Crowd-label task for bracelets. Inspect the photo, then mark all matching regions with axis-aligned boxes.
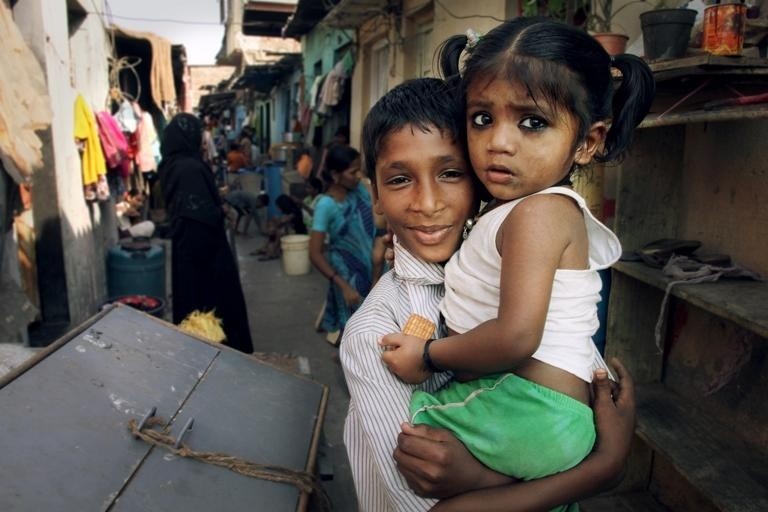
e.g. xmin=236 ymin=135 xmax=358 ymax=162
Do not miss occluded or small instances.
xmin=329 ymin=271 xmax=338 ymax=283
xmin=419 ymin=337 xmax=446 ymax=375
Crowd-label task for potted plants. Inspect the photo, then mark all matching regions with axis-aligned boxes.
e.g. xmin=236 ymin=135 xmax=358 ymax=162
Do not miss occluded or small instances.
xmin=639 ymin=0 xmax=699 ymax=63
xmin=586 ymin=0 xmax=639 ymax=55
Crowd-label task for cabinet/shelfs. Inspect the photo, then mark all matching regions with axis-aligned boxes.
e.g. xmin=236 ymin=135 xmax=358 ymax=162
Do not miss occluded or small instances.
xmin=602 ymin=105 xmax=768 ymax=512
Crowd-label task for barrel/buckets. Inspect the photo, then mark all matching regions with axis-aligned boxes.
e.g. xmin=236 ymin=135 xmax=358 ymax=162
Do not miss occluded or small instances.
xmin=278 ymin=234 xmax=311 ymax=276
xmin=109 ymin=237 xmax=167 ymax=300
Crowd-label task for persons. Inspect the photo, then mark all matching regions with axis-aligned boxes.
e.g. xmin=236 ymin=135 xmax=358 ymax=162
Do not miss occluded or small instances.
xmin=376 ymin=13 xmax=657 ymax=511
xmin=107 ymin=106 xmax=354 ymax=264
xmin=303 ymin=142 xmax=385 ymax=342
xmin=153 ymin=113 xmax=257 ymax=357
xmin=337 ymin=75 xmax=640 ymax=512
